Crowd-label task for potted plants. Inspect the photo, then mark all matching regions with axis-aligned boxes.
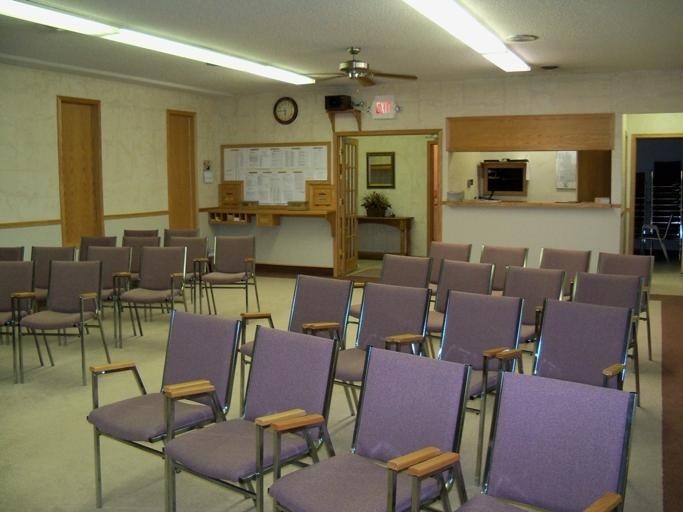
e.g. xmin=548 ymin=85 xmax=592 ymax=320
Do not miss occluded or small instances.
xmin=358 ymin=190 xmax=391 ymax=217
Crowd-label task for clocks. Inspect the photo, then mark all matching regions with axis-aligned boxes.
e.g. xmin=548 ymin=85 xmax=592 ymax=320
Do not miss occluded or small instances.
xmin=272 ymin=97 xmax=298 ymax=125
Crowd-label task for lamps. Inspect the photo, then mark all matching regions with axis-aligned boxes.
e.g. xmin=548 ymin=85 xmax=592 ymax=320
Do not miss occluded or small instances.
xmin=0 ymin=0 xmax=315 ymax=88
xmin=398 ymin=0 xmax=533 ymax=76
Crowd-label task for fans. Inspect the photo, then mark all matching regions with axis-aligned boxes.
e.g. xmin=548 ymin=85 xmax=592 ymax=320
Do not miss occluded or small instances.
xmin=298 ymin=47 xmax=418 ymax=88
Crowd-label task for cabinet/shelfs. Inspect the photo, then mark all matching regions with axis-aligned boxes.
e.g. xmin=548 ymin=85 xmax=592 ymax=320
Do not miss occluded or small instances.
xmin=634 ymin=195 xmax=644 ymax=237
xmin=356 ymin=216 xmax=414 ymax=257
xmin=650 ymin=183 xmax=682 ymax=239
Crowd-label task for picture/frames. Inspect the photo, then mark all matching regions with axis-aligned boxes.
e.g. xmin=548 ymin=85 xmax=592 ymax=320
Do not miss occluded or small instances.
xmin=366 ymin=152 xmax=394 ymax=188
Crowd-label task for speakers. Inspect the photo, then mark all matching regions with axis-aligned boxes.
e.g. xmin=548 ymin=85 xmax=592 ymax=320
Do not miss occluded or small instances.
xmin=324 ymin=95 xmax=352 ymax=111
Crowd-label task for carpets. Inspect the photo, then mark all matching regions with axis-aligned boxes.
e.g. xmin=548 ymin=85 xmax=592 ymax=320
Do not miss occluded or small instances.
xmin=0 ymin=267 xmax=666 ymax=511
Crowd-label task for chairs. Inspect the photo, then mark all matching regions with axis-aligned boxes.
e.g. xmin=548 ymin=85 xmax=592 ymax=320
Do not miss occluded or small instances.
xmin=1 ymin=228 xmax=260 ymax=385
xmin=88 ymin=240 xmax=656 ymax=511
xmin=637 ymin=214 xmax=673 ymax=263
